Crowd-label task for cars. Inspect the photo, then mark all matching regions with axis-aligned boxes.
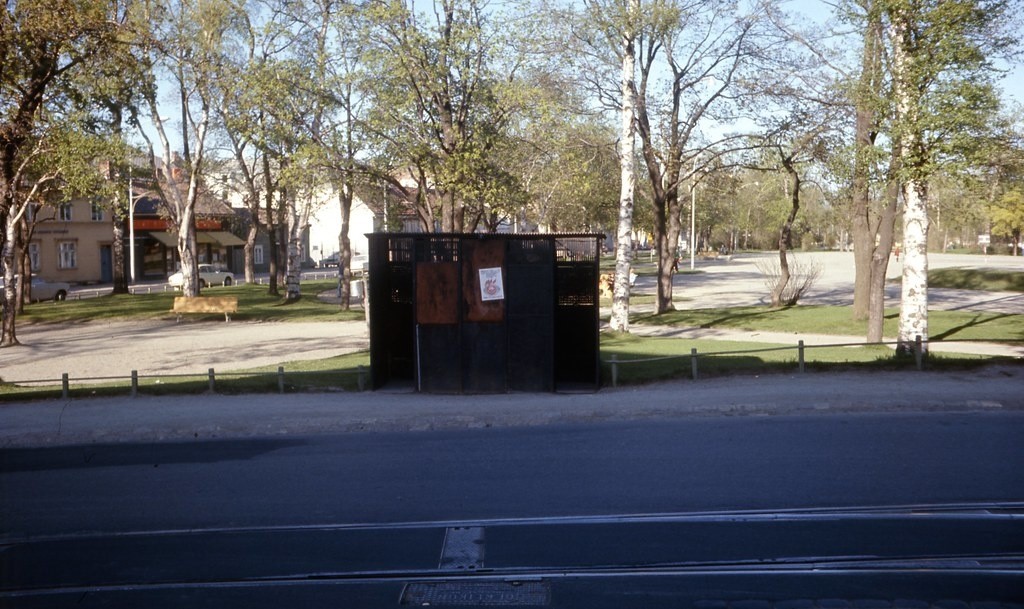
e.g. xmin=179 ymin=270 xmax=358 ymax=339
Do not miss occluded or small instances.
xmin=0 ymin=273 xmax=71 ymax=307
xmin=168 ymin=263 xmax=237 ymax=291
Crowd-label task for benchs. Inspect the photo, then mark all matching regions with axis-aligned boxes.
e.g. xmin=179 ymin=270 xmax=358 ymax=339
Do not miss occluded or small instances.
xmin=169 ymin=296 xmax=238 ymax=325
xmin=697 ymin=252 xmax=719 ymax=260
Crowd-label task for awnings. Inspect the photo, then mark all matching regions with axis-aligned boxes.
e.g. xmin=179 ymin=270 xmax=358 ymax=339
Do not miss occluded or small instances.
xmin=149 ymin=229 xmax=246 ymax=248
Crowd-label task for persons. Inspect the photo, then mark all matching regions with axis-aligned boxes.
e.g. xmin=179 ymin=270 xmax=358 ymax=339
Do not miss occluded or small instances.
xmin=894 ymin=246 xmax=900 ymax=261
xmin=720 ymin=244 xmax=727 ymax=255
xmin=671 ymin=256 xmax=683 ymax=273
